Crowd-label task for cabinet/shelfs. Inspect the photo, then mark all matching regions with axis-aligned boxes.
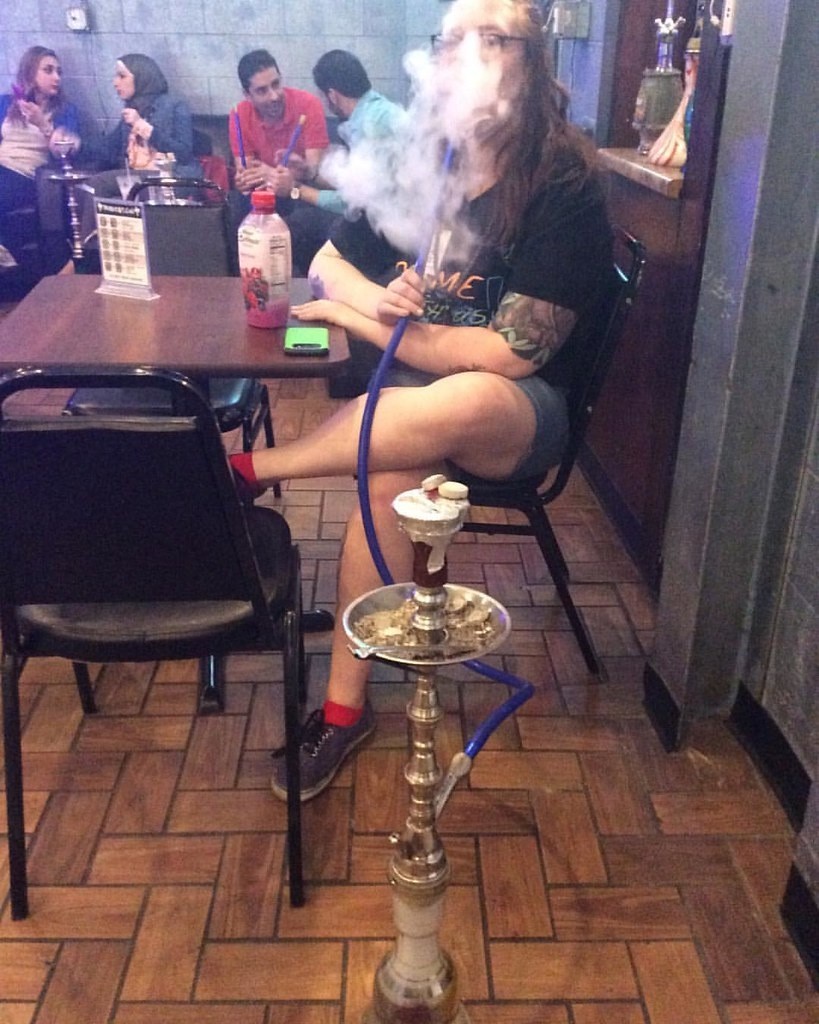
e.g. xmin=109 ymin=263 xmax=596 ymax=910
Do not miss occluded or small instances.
xmin=577 ymin=0 xmax=731 ymax=581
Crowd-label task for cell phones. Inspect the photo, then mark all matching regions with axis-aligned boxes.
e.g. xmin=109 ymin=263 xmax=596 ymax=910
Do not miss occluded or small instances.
xmin=283 ymin=327 xmax=331 ymax=355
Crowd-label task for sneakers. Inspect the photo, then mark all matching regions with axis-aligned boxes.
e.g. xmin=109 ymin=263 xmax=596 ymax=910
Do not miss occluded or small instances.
xmin=269 ymin=696 xmax=378 ymax=803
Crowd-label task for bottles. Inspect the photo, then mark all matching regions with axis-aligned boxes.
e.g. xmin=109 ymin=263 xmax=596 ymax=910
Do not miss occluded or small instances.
xmin=237 ymin=190 xmax=292 ymax=330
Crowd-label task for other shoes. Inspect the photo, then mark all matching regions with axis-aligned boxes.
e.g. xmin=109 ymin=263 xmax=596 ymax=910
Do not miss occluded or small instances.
xmin=0 ymin=246 xmax=19 ymax=279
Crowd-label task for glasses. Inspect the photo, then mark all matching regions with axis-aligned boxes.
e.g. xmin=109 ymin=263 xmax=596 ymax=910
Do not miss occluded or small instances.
xmin=430 ymin=31 xmax=529 ymax=63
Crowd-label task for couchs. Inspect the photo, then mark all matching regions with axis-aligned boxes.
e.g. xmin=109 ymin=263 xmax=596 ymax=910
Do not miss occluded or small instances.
xmin=0 ymin=165 xmax=67 ymax=301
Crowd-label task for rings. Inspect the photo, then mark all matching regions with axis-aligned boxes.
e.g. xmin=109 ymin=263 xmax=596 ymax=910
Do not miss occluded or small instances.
xmin=268 ymin=182 xmax=274 ymax=188
xmin=261 ymin=177 xmax=264 ymax=185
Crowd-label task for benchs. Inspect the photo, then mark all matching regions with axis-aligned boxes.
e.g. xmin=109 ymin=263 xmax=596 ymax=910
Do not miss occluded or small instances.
xmin=187 ymin=113 xmax=347 ymax=170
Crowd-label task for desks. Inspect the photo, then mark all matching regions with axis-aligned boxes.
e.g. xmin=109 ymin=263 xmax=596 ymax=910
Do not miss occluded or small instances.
xmin=0 ymin=275 xmax=351 ymax=712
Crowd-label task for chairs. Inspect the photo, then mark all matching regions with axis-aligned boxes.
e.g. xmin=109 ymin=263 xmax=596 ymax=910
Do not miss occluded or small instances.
xmin=0 ymin=179 xmax=646 ymax=920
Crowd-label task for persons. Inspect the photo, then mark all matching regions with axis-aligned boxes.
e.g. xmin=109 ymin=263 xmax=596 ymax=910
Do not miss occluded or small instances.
xmin=0 ymin=45 xmax=79 ymax=269
xmin=227 ymin=0 xmax=614 ymax=805
xmin=265 ymin=46 xmax=416 ymax=278
xmin=60 ymin=53 xmax=193 ymax=277
xmin=222 ymin=46 xmax=332 ymax=279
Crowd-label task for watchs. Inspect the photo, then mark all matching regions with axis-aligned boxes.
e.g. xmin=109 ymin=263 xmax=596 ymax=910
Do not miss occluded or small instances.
xmin=290 ymin=182 xmax=304 ymax=200
